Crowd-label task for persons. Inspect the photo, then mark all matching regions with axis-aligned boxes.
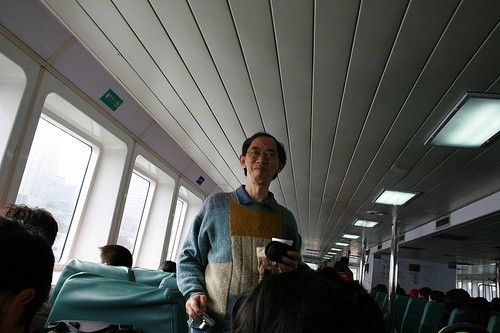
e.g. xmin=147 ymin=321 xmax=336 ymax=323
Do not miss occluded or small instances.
xmin=176 ymin=132 xmax=302 ymax=333
xmin=317 ymin=257 xmax=353 ymax=281
xmin=370 ymin=283 xmax=500 ymax=310
xmin=0 ymin=202 xmax=177 ymax=333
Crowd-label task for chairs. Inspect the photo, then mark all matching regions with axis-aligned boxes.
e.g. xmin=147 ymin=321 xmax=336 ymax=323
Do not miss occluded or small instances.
xmin=46 ymin=258 xmax=189 ymax=333
xmin=370 ymin=290 xmax=500 ymax=333
